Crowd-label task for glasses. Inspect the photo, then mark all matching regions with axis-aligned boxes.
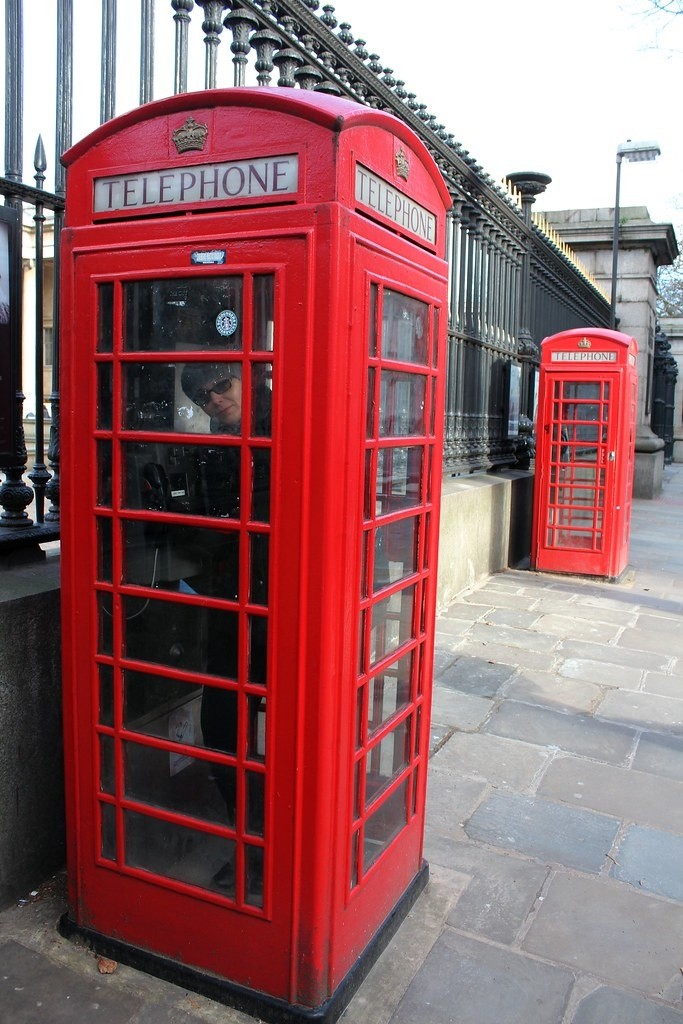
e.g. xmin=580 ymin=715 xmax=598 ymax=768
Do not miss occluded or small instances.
xmin=193 ymin=376 xmax=235 ymax=407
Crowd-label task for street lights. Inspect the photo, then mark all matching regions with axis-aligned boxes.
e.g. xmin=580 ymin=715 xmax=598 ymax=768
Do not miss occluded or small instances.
xmin=609 ymin=138 xmax=662 ymax=330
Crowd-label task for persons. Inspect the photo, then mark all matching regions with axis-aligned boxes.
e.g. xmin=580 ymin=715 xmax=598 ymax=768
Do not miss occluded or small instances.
xmin=140 ymin=348 xmax=274 ymax=895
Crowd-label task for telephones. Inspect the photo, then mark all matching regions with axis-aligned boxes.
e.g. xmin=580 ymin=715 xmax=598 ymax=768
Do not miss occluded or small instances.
xmin=107 ymin=448 xmax=213 ymax=585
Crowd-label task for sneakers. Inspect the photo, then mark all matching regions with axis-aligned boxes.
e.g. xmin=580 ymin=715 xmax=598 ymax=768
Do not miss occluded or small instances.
xmin=212 ymin=844 xmax=263 ymax=895
